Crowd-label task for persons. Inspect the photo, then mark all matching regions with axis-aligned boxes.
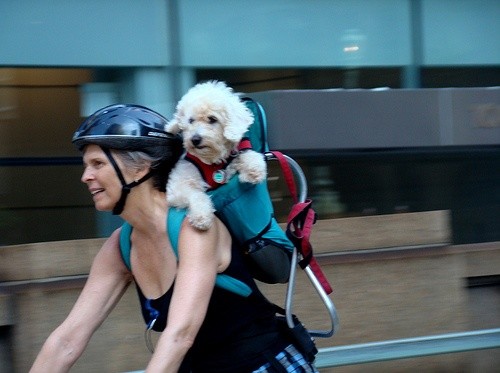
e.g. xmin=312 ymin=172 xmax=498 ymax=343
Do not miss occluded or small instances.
xmin=27 ymin=100 xmax=323 ymax=373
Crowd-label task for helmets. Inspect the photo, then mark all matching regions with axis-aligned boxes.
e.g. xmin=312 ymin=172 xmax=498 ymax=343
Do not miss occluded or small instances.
xmin=72 ymin=104 xmax=183 ymax=152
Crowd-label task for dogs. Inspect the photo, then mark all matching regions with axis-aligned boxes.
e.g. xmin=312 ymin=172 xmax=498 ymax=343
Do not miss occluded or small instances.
xmin=164 ymin=78 xmax=268 ymax=232
